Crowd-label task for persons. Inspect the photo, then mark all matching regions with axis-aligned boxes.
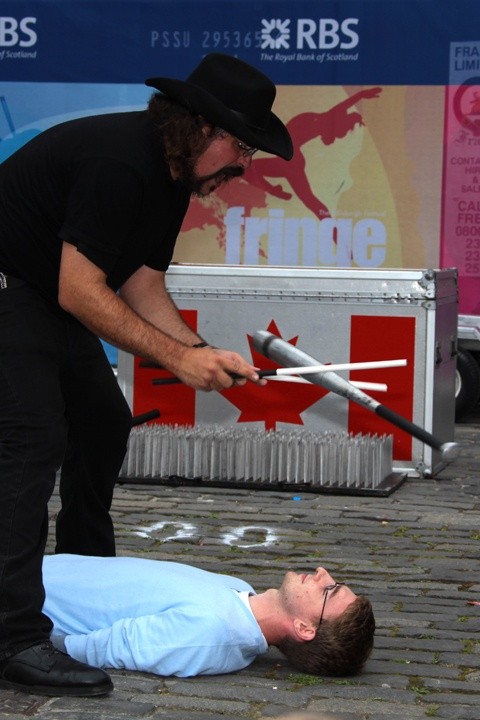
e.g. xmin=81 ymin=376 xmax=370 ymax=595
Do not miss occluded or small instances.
xmin=0 ymin=52 xmax=293 ymax=697
xmin=41 ymin=552 xmax=376 ymax=679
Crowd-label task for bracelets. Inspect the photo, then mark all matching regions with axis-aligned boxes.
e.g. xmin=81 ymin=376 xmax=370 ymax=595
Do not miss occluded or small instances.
xmin=193 ymin=342 xmax=208 ymax=348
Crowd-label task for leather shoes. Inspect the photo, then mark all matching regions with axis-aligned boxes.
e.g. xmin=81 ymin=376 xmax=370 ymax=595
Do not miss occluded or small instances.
xmin=0 ymin=641 xmax=114 ymax=696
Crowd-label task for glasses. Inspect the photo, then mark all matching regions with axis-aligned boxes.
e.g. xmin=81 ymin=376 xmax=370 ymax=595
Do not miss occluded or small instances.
xmin=231 ymin=135 xmax=258 ymax=157
xmin=305 ymin=582 xmax=345 ymax=645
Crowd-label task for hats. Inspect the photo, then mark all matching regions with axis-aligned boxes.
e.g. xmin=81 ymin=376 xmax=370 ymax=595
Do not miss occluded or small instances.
xmin=144 ymin=54 xmax=293 ymax=161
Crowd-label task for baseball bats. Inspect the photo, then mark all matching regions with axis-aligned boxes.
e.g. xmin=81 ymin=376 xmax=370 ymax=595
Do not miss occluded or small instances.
xmin=253 ymin=328 xmax=461 ymax=465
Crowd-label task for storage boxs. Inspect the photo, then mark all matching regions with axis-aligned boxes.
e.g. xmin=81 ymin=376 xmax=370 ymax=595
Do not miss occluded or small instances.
xmin=117 ymin=262 xmax=459 ymax=479
